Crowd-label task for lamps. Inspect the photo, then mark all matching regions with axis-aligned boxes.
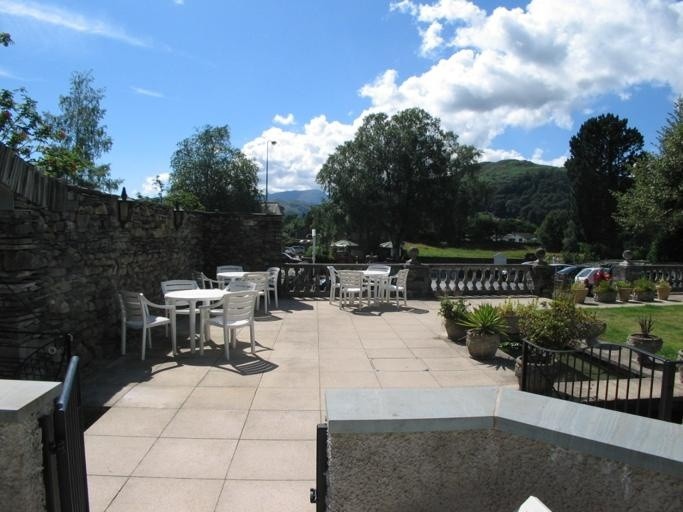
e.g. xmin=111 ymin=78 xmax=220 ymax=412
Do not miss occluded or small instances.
xmin=117 ymin=187 xmax=130 ymax=229
xmin=173 ymin=202 xmax=184 ymax=230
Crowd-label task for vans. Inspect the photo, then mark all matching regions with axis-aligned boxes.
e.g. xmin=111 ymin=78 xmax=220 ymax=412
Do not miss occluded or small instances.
xmin=292 ymin=246 xmax=305 ymax=254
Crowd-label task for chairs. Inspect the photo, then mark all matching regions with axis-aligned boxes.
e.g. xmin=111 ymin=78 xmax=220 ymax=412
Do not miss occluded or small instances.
xmin=118 ymin=262 xmax=409 ymax=362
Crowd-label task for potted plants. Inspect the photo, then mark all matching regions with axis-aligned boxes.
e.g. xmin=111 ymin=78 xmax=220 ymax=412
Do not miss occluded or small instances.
xmin=570 ymin=279 xmax=589 ymax=303
xmin=615 ymin=280 xmax=634 ymax=301
xmin=655 ymin=278 xmax=672 ymax=299
xmin=626 ymin=313 xmax=663 ymax=365
xmin=594 ymin=280 xmax=617 ymax=302
xmin=437 ymin=293 xmax=607 ymax=393
xmin=633 ymin=275 xmax=656 ymax=301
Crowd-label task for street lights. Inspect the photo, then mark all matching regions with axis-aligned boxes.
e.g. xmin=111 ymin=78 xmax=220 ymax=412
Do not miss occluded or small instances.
xmin=265 ymin=139 xmax=276 ymax=206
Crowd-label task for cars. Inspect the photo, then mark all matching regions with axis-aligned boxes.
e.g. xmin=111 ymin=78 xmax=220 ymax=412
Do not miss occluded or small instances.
xmin=547 ymin=262 xmax=620 ymax=297
xmin=284 ymin=247 xmax=295 ymax=257
